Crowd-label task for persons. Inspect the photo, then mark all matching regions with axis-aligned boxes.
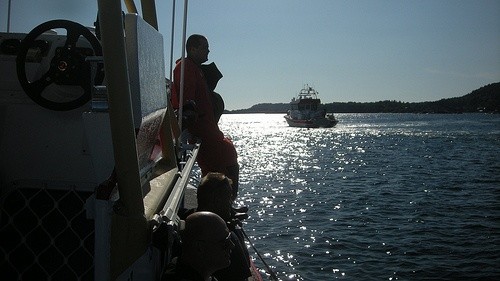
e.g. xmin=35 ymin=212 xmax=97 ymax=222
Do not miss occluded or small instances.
xmin=163 ymin=172 xmax=252 ymax=281
xmin=172 ymin=34 xmax=249 ymax=219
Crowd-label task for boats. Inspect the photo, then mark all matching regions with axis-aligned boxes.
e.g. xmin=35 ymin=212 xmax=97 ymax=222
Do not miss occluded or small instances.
xmin=283 ymin=82 xmax=339 ymax=128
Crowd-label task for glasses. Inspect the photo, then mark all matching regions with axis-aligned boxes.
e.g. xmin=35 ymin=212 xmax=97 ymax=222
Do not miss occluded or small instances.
xmin=214 ymin=175 xmax=229 ymax=189
xmin=196 ymin=232 xmax=232 ymax=249
